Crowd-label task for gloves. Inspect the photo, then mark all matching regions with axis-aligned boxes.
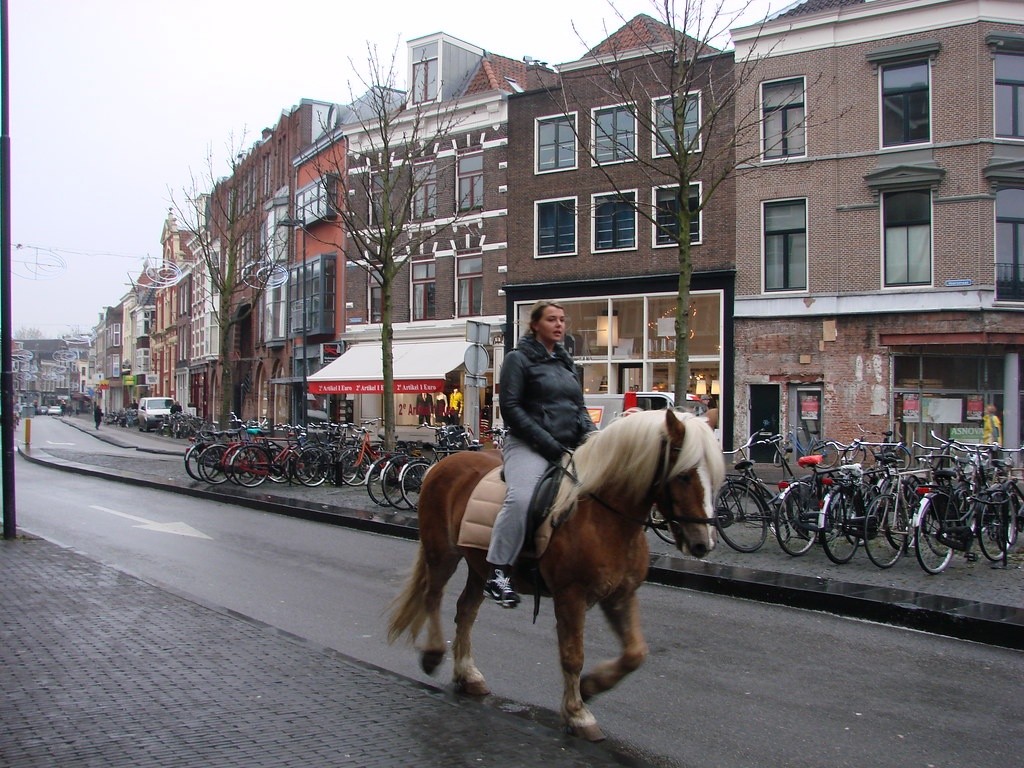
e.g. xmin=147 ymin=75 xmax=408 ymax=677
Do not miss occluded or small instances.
xmin=544 ymin=438 xmax=567 ymax=461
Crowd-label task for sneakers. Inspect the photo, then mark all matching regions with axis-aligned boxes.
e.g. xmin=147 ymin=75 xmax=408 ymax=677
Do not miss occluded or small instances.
xmin=482 ymin=569 xmax=521 ymax=608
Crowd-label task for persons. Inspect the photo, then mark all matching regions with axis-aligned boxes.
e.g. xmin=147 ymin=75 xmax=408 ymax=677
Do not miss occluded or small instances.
xmin=94 ymin=406 xmax=103 ymax=429
xmin=61 ymin=404 xmax=73 ymax=417
xmin=982 ymin=405 xmax=1003 ymax=448
xmin=170 ymin=401 xmax=182 ymax=414
xmin=482 ymin=301 xmax=599 ymax=606
xmin=416 ymin=389 xmax=463 ymax=427
xmin=132 ymin=400 xmax=138 ymax=409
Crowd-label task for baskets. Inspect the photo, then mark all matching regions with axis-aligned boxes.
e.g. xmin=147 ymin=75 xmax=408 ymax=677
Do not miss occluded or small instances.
xmin=440 ymin=429 xmax=465 ymax=455
xmin=229 ymin=420 xmax=242 ymax=429
xmin=309 ymin=432 xmax=327 ymax=442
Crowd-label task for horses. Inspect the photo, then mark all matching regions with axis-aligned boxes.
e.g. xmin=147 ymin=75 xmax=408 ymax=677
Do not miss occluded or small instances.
xmin=383 ymin=407 xmax=725 ymax=741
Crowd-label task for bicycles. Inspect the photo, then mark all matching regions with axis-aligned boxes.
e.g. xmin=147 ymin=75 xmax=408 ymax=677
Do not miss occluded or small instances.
xmin=103 ymin=406 xmax=140 ymax=429
xmin=156 ymin=404 xmax=510 ymax=513
xmin=647 ymin=423 xmax=1024 ymax=575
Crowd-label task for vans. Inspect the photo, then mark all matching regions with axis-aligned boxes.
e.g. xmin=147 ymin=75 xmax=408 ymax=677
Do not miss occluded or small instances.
xmin=138 ymin=396 xmax=177 ymax=432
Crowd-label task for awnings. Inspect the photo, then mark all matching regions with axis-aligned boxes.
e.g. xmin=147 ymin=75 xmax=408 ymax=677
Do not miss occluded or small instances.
xmin=307 ymin=342 xmax=482 ymax=395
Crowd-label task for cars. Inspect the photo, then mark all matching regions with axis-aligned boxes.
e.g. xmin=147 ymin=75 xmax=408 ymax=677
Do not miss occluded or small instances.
xmin=37 ymin=406 xmax=48 ymax=415
xmin=48 ymin=405 xmax=63 ymax=416
